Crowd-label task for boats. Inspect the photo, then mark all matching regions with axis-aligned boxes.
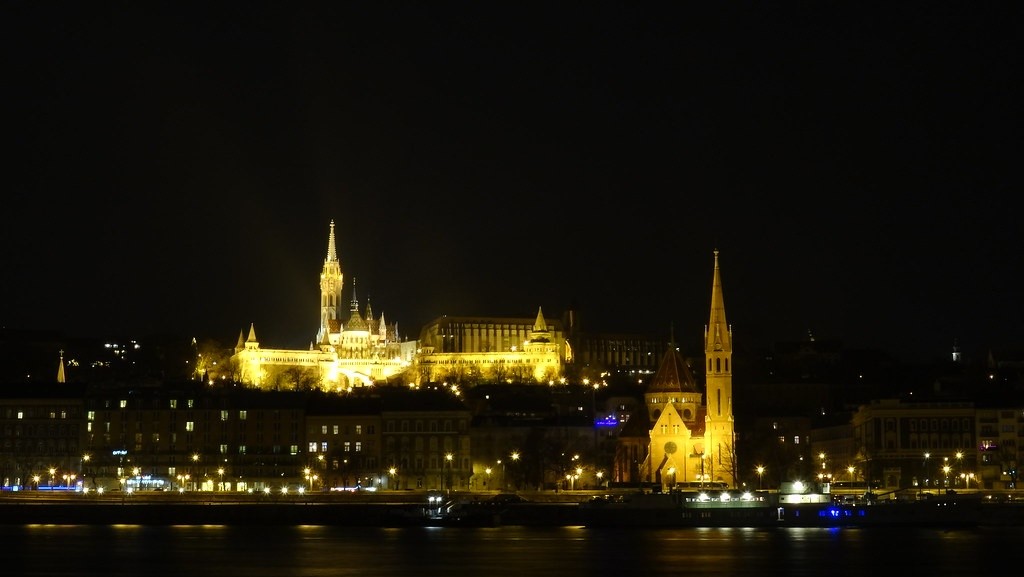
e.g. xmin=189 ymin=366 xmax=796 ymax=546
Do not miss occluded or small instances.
xmin=826 ymin=483 xmax=985 ymax=528
xmin=583 ymin=484 xmax=778 ymax=525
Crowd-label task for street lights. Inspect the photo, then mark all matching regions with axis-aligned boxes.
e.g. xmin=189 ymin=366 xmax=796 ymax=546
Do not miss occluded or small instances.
xmin=219 ymin=470 xmax=223 ymax=490
xmin=194 ymin=456 xmax=199 ymax=491
xmin=441 ymin=455 xmax=452 ymax=490
